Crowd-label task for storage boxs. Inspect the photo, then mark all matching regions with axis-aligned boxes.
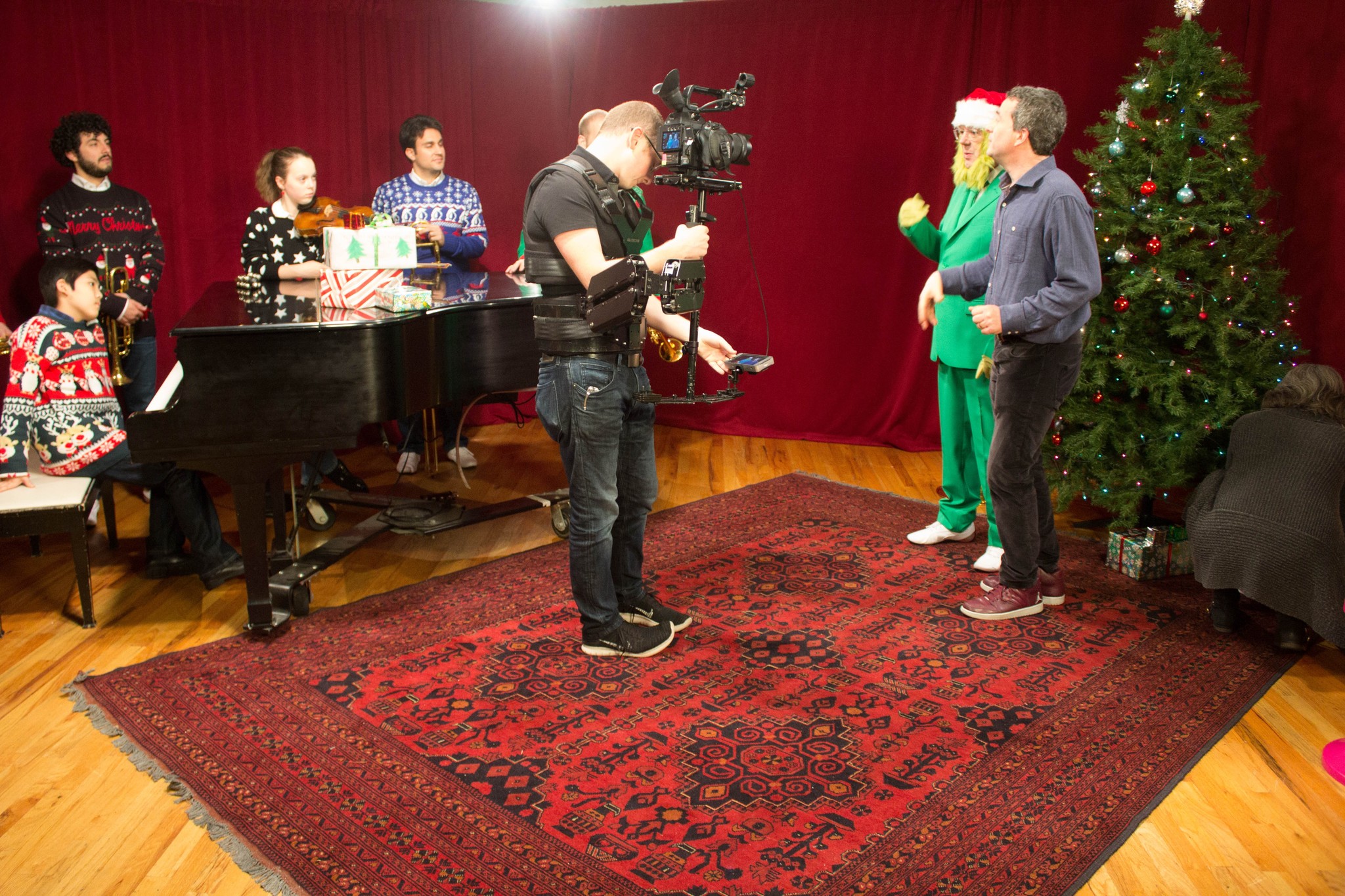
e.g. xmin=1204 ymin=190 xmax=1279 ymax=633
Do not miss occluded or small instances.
xmin=375 ymin=285 xmax=432 ymax=313
xmin=323 ymin=226 xmax=417 ymax=271
xmin=320 ymin=269 xmax=404 ymax=310
xmin=1105 ymin=526 xmax=1193 ymax=581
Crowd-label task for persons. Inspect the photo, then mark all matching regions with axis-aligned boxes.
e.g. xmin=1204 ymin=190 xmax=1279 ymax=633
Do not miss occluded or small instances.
xmin=916 ymin=85 xmax=1104 ymax=621
xmin=0 ymin=256 xmax=247 ymax=590
xmin=0 ymin=312 xmax=13 ymax=340
xmin=504 ymin=108 xmax=656 ymax=538
xmin=896 ymin=88 xmax=1005 ymax=573
xmin=523 ymin=100 xmax=737 ymax=658
xmin=36 ymin=110 xmax=166 ymax=527
xmin=371 ymin=115 xmax=489 ymax=474
xmin=1181 ymin=362 xmax=1345 ymax=654
xmin=241 ymin=146 xmax=367 ymax=511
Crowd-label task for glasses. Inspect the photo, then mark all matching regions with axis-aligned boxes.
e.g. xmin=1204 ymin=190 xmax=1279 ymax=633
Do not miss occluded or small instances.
xmin=953 ymin=127 xmax=980 ymax=141
xmin=632 ymin=127 xmax=666 ymax=176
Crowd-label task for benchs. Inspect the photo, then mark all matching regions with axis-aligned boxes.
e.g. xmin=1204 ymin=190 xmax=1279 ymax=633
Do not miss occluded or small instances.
xmin=0 ymin=445 xmax=118 ymax=638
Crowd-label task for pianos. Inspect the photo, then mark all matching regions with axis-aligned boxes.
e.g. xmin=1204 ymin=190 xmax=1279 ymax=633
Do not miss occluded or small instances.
xmin=127 ymin=270 xmax=545 ymax=637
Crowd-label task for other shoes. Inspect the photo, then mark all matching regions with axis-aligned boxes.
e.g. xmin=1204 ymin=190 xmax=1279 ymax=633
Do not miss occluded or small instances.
xmin=327 ymin=461 xmax=370 ymax=493
xmin=85 ymin=499 xmax=100 ymax=525
xmin=145 ymin=547 xmax=195 ymax=578
xmin=203 ymin=553 xmax=243 ymax=589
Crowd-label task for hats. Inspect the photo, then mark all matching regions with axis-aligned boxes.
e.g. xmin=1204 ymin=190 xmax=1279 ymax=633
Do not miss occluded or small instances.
xmin=952 ymin=88 xmax=1008 ymax=130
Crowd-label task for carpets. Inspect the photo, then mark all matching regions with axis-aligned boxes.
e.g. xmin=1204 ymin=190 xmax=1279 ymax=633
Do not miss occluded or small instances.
xmin=58 ymin=469 xmax=1314 ymax=896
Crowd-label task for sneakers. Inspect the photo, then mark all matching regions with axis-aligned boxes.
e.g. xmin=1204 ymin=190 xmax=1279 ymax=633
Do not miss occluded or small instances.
xmin=396 ymin=451 xmax=422 ymax=474
xmin=446 ymin=445 xmax=478 ymax=471
xmin=979 ymin=563 xmax=1066 ymax=604
xmin=907 ymin=519 xmax=976 ymax=544
xmin=580 ymin=619 xmax=675 ymax=659
xmin=960 ymin=580 xmax=1045 ymax=621
xmin=973 ymin=545 xmax=1006 ymax=571
xmin=616 ymin=587 xmax=693 ymax=632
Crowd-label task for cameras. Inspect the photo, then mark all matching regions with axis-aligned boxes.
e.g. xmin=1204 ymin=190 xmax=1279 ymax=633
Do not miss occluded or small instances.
xmin=725 ymin=353 xmax=774 ymax=372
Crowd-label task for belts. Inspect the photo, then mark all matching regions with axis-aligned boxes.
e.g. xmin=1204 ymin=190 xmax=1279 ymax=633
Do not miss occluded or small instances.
xmin=540 ymin=352 xmax=643 ymax=368
